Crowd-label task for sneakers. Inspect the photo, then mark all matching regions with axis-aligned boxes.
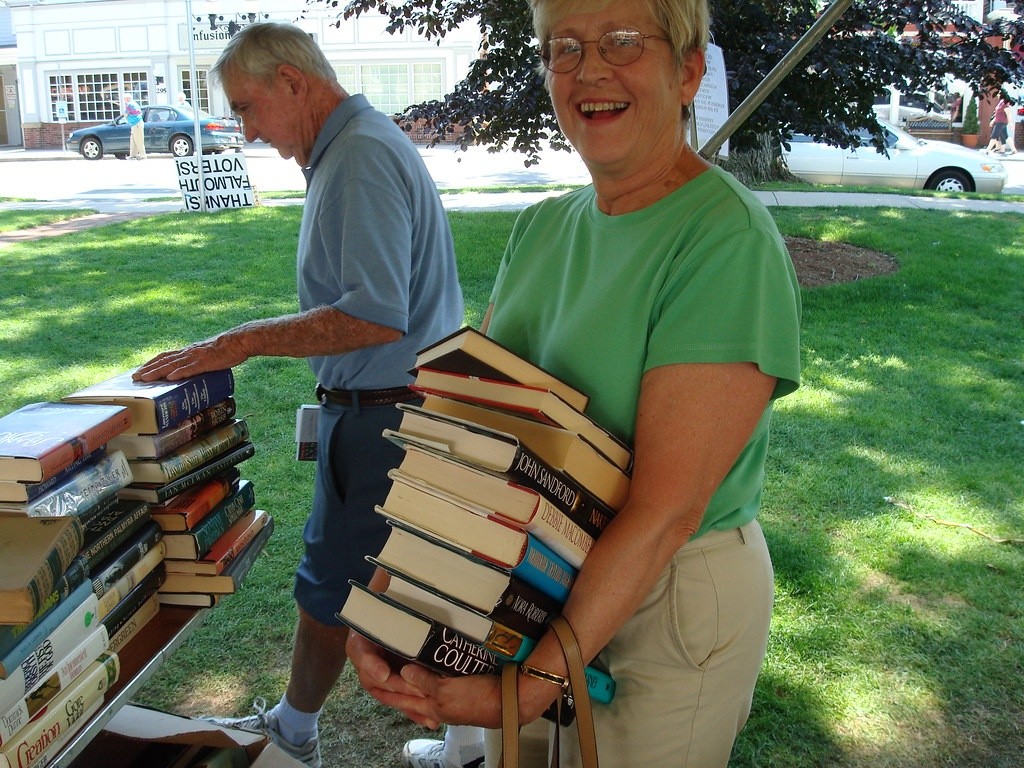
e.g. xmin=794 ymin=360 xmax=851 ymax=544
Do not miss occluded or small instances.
xmin=197 ymin=695 xmax=324 ymax=768
xmin=401 ymin=738 xmax=444 ymax=768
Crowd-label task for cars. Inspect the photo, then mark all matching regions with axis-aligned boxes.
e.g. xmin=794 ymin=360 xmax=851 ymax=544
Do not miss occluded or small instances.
xmin=715 ymin=112 xmax=1007 ymax=197
xmin=66 ymin=104 xmax=242 ymax=161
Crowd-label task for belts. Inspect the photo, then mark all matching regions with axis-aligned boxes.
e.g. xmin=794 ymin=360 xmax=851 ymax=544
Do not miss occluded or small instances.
xmin=324 ymin=386 xmax=421 ymax=405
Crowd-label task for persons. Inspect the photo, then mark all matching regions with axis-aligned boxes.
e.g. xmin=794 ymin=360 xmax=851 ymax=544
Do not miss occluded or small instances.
xmin=979 ymin=89 xmax=1018 ymax=155
xmin=486 ymin=0 xmax=801 ymax=768
xmin=123 ymin=93 xmax=147 ymax=160
xmin=136 ymin=21 xmax=490 ymax=767
xmin=175 ymin=92 xmax=191 ymax=106
xmin=943 ymin=90 xmax=962 ymax=123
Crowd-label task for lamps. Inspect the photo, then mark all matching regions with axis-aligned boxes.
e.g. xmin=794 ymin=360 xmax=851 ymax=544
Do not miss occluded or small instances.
xmin=192 ymin=12 xmax=201 ymax=30
xmin=248 ymin=11 xmax=269 ymax=23
xmin=228 ymin=12 xmax=246 ymax=38
xmin=208 ymin=14 xmax=224 ymax=30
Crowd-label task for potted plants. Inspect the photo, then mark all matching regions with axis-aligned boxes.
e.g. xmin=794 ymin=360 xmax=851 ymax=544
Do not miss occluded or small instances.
xmin=959 ymin=96 xmax=980 ymax=149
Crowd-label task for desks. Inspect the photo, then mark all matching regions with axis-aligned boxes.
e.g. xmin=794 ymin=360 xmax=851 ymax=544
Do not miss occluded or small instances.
xmin=44 ymin=594 xmax=224 ymax=768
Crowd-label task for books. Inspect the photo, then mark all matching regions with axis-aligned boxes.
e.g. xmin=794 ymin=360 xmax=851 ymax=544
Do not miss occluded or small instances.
xmin=0 ymin=363 xmax=277 ymax=768
xmin=334 ymin=324 xmax=635 ymax=727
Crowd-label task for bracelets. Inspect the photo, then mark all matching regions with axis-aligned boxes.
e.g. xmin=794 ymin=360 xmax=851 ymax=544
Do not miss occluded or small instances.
xmin=519 ymin=665 xmax=570 ymax=689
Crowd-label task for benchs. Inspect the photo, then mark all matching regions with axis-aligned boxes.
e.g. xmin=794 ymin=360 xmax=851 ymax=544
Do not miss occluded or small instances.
xmin=906 ymin=116 xmax=954 ymax=142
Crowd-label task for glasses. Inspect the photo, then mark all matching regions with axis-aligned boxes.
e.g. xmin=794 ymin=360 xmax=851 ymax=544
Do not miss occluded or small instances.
xmin=537 ymin=27 xmax=671 ymax=73
